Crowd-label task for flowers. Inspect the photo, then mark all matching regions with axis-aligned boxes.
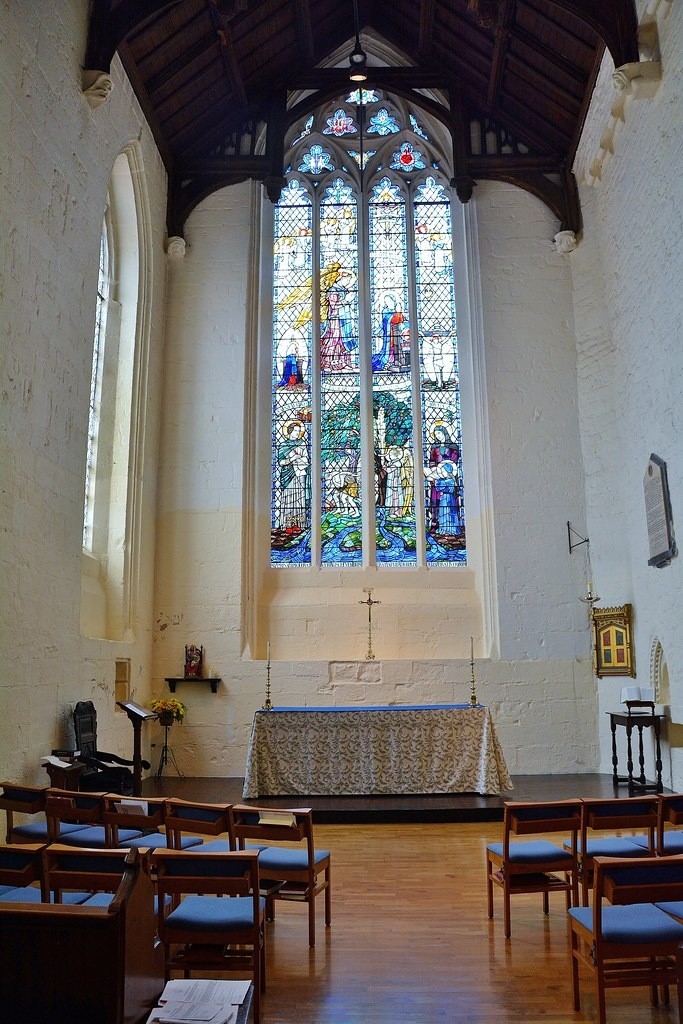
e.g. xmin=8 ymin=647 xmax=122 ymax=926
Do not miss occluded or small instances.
xmin=145 ymin=692 xmax=188 ymax=720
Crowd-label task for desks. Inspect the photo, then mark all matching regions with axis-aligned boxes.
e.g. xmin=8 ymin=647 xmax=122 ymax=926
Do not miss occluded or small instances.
xmin=606 ymin=711 xmax=667 ymax=794
xmin=142 ymin=980 xmax=255 ymax=1024
xmin=241 ymin=703 xmax=516 ymax=801
xmin=41 ymin=760 xmax=87 ymax=792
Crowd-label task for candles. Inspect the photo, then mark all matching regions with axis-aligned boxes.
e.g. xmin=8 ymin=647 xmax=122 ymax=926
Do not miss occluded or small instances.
xmin=588 ymin=582 xmax=592 ymax=592
xmin=469 ymin=636 xmax=473 ymax=663
xmin=267 ymin=640 xmax=271 ymax=667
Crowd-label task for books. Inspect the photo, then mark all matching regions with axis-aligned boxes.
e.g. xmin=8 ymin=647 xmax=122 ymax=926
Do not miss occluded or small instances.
xmin=41 ymin=749 xmax=81 ymax=769
xmin=620 ymin=686 xmax=656 ymax=704
xmin=249 ymin=879 xmax=318 ymax=901
xmin=114 ymin=798 xmax=149 ymax=816
xmin=117 ymin=701 xmax=158 ymax=720
xmin=258 ymin=812 xmax=297 ymax=829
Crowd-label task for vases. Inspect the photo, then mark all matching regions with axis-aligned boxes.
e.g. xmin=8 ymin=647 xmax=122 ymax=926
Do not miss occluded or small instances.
xmin=159 ymin=712 xmax=175 ymax=726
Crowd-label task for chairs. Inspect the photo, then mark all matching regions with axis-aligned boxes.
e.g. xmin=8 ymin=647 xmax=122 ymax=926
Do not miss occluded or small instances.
xmin=485 ymin=792 xmax=683 ymax=1024
xmin=348 ymin=0 xmax=368 ymax=82
xmin=70 ymin=699 xmax=151 ymax=797
xmin=0 ymin=776 xmax=333 ymax=995
